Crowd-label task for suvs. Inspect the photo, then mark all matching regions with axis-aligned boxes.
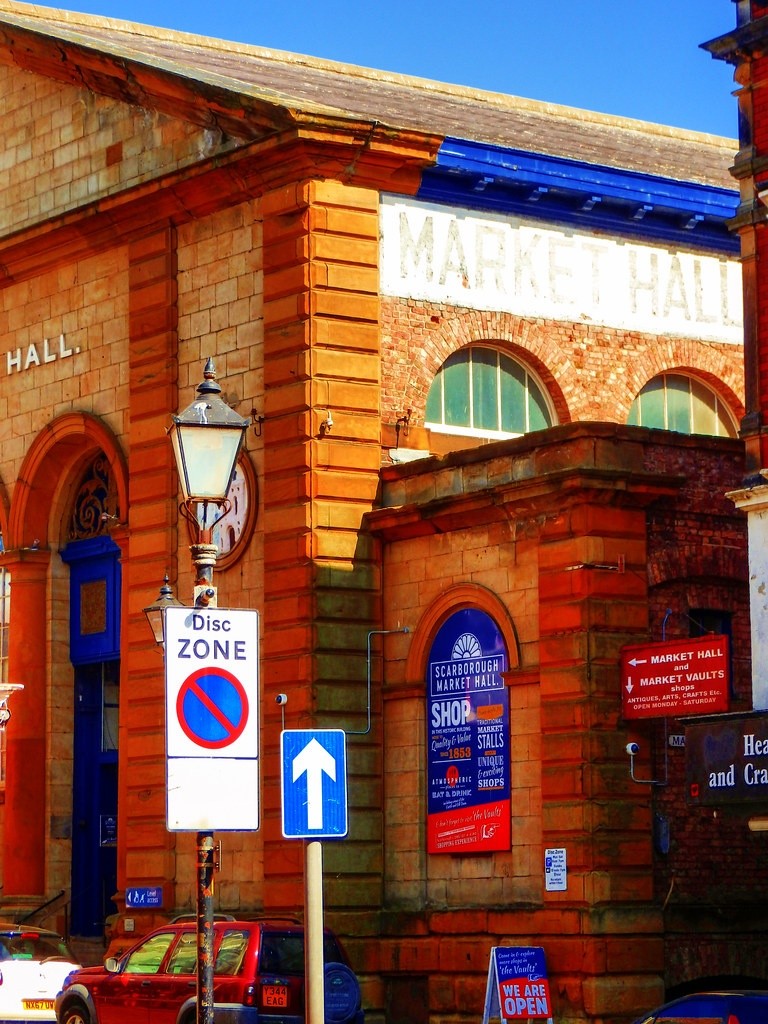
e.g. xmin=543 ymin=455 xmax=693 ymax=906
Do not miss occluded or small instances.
xmin=54 ymin=913 xmax=365 ymax=1024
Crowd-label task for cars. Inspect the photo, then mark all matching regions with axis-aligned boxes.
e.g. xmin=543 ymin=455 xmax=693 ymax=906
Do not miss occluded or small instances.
xmin=0 ymin=923 xmax=83 ymax=1021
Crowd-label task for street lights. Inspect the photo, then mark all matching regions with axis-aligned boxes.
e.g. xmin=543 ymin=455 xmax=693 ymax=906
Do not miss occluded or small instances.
xmin=160 ymin=358 xmax=249 ymax=1024
